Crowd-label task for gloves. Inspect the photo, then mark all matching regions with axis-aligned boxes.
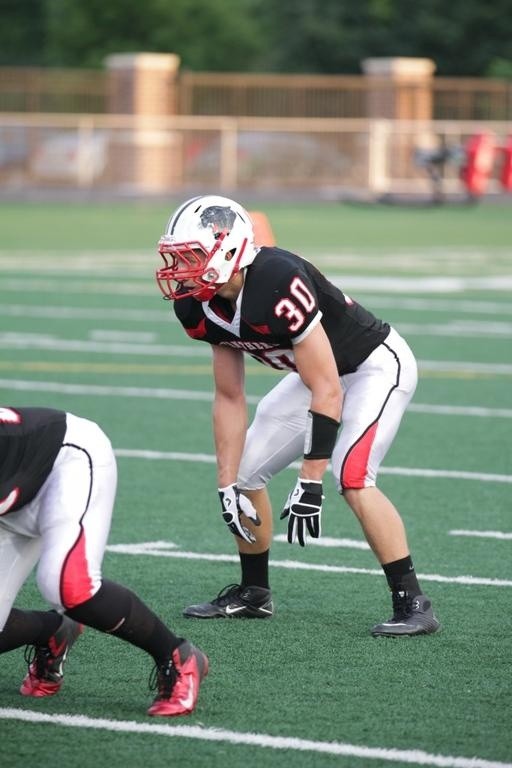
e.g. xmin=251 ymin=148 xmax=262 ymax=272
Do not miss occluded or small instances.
xmin=280 ymin=476 xmax=323 ymax=548
xmin=219 ymin=483 xmax=260 ymax=543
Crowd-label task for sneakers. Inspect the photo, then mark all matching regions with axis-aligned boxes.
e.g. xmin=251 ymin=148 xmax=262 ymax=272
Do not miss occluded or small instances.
xmin=147 ymin=638 xmax=208 ymax=717
xmin=183 ymin=584 xmax=274 ymax=619
xmin=372 ymin=596 xmax=440 ymax=637
xmin=20 ymin=609 xmax=82 ymax=697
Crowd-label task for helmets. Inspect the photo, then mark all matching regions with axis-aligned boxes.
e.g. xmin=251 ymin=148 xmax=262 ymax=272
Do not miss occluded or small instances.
xmin=158 ymin=195 xmax=258 ymax=298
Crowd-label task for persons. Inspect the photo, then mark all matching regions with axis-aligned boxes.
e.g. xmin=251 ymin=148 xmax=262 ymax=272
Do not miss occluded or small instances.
xmin=155 ymin=195 xmax=441 ymax=636
xmin=0 ymin=406 xmax=209 ymax=717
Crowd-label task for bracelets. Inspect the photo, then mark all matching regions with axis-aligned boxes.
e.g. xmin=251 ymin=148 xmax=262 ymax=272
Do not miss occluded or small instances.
xmin=304 ymin=409 xmax=340 ymax=459
